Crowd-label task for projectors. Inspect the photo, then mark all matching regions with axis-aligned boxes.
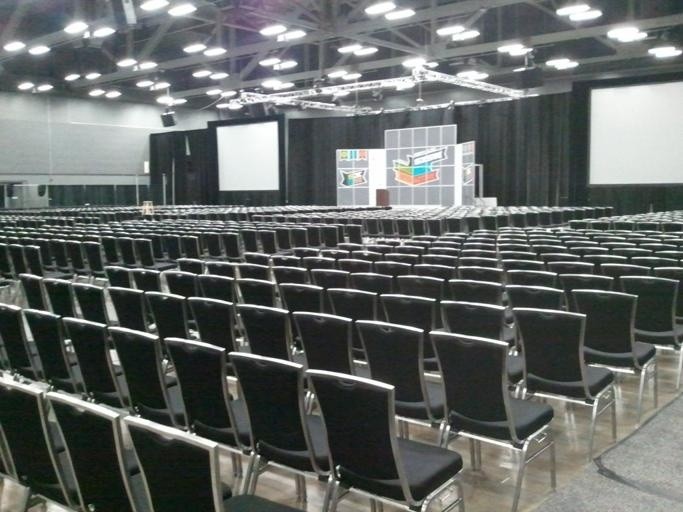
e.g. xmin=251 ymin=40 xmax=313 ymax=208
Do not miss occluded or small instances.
xmin=521 ymin=67 xmax=543 ymax=88
xmin=160 ymin=110 xmax=177 ymax=127
